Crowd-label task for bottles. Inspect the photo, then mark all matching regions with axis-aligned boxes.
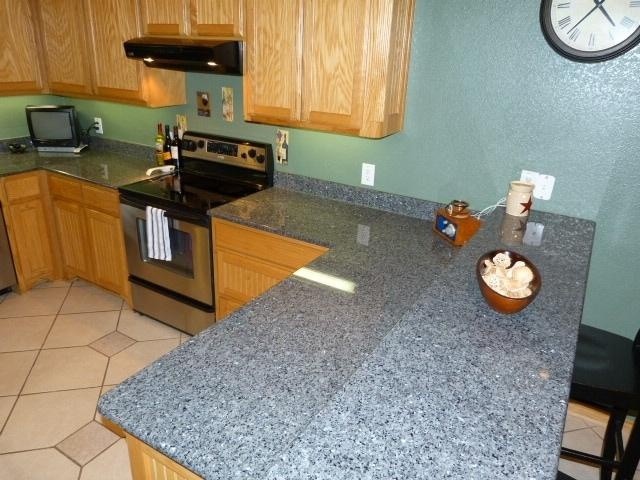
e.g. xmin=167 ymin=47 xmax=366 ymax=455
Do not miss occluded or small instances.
xmin=163 ymin=125 xmax=173 ymax=165
xmin=154 ymin=123 xmax=165 ymax=165
xmin=171 ymin=126 xmax=184 ymax=168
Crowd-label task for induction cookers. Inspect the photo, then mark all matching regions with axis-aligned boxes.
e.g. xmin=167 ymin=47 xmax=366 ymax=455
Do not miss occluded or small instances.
xmin=113 ymin=158 xmax=267 ymax=216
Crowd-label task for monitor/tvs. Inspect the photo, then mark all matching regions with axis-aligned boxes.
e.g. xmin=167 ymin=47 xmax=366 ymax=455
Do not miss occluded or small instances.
xmin=24 ymin=104 xmax=80 ymax=152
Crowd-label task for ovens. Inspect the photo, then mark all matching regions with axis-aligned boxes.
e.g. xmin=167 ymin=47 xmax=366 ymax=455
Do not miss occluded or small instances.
xmin=117 ymin=195 xmax=216 ymax=337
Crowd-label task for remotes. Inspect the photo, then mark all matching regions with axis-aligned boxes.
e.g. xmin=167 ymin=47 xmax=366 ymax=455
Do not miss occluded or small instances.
xmin=74 ymin=145 xmax=89 ymax=155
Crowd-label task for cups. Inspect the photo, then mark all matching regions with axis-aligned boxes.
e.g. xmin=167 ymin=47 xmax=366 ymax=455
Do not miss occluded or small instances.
xmin=505 ymin=181 xmax=534 ymax=217
xmin=499 ymin=213 xmax=527 ymax=247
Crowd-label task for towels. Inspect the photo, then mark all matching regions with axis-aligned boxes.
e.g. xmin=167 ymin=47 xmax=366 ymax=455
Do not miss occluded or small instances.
xmin=145 ymin=205 xmax=174 ymax=262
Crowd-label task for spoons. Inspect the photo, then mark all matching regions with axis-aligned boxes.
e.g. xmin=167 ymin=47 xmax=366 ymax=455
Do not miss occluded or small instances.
xmin=146 ymin=166 xmax=175 ymax=176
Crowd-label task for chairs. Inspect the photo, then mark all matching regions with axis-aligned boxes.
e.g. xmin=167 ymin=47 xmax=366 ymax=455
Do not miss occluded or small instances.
xmin=556 ymin=324 xmax=639 ymax=480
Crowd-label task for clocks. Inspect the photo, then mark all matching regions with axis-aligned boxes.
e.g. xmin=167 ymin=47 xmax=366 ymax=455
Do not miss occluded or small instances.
xmin=537 ymin=0 xmax=640 ymax=66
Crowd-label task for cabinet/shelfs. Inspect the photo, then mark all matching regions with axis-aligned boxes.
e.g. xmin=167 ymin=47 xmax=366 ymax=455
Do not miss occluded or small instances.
xmin=143 ymin=2 xmax=242 ymax=40
xmin=38 ymin=2 xmax=186 ymax=108
xmin=46 ymin=171 xmax=134 ymax=311
xmin=211 ymin=217 xmax=330 ymax=322
xmin=0 ymin=170 xmax=62 ymax=295
xmin=3 ymin=2 xmax=46 ymax=97
xmin=244 ymin=0 xmax=412 ymax=139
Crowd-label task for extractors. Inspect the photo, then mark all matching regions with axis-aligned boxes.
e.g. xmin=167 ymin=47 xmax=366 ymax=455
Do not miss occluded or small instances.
xmin=122 ymin=36 xmax=242 ymax=76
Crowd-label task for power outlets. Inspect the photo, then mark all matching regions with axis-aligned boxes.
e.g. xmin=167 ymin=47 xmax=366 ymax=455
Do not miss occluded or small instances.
xmin=93 ymin=116 xmax=104 ymax=135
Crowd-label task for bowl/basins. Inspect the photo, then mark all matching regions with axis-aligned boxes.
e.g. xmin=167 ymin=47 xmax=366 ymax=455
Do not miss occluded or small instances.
xmin=7 ymin=143 xmax=26 ymax=153
xmin=477 ymin=250 xmax=542 ymax=315
